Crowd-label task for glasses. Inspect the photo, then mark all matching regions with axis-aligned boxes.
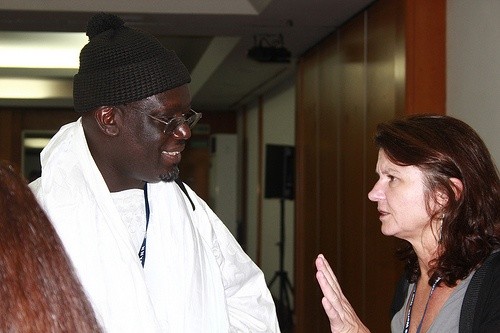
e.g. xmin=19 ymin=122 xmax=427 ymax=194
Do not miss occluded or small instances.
xmin=120 ymin=102 xmax=202 ymax=134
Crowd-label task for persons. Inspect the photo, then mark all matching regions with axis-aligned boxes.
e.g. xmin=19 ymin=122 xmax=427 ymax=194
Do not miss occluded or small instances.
xmin=27 ymin=11 xmax=281 ymax=333
xmin=315 ymin=113 xmax=500 ymax=333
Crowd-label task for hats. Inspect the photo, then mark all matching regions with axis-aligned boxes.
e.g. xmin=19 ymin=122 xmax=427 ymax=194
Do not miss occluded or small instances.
xmin=73 ymin=12 xmax=191 ymax=114
